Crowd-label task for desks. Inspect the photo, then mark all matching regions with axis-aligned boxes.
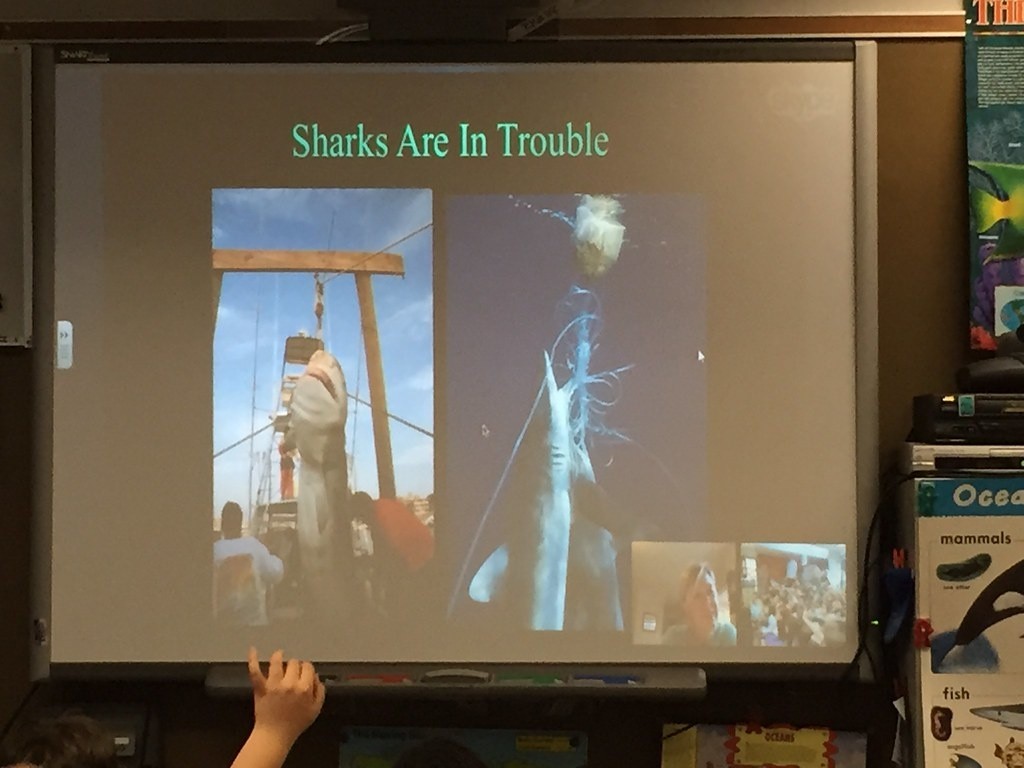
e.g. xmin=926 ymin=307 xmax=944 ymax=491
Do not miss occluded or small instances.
xmin=204 ymin=661 xmax=709 ymax=768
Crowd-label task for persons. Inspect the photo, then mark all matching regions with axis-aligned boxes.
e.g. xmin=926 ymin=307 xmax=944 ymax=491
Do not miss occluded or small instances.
xmin=0 ymin=644 xmax=324 ymax=768
xmin=213 ymin=488 xmax=437 ymax=625
xmin=659 ymin=560 xmax=847 ymax=647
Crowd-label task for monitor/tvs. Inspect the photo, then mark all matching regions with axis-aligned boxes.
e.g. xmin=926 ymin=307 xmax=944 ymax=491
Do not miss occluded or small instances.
xmin=136 ymin=687 xmax=663 ymax=768
